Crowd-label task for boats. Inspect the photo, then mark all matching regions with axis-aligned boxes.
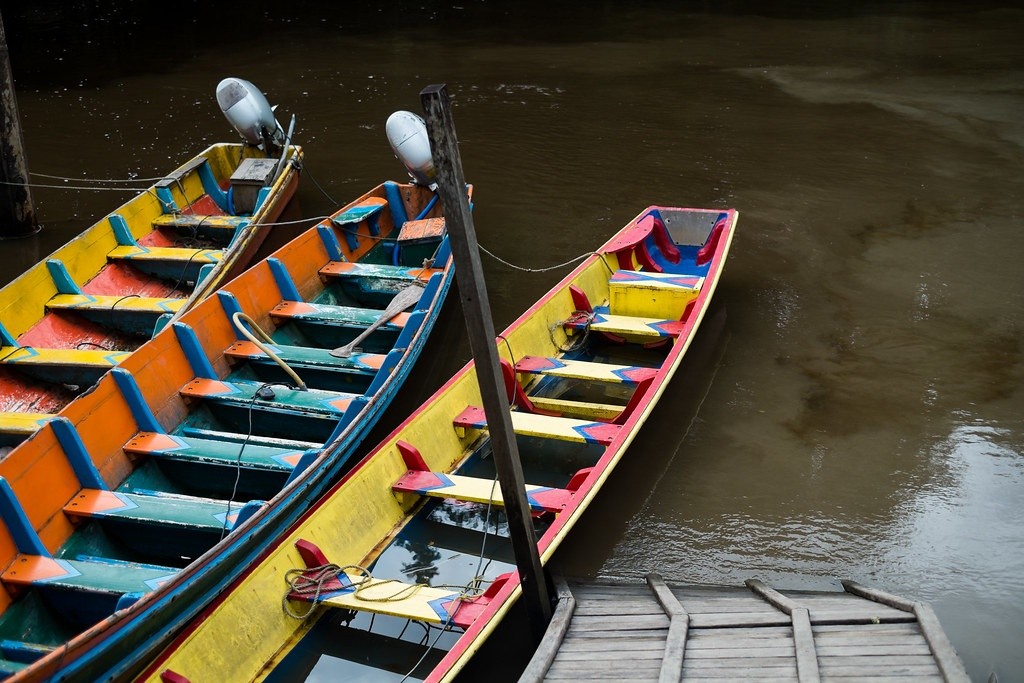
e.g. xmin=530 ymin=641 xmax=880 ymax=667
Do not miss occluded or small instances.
xmin=97 ymin=203 xmax=742 ymax=680
xmin=0 ymin=110 xmax=473 ymax=682
xmin=0 ymin=76 xmax=305 ymax=463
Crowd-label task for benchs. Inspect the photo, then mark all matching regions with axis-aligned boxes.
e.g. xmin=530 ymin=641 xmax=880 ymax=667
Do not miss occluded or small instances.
xmin=223 ymin=338 xmax=388 ymax=376
xmin=453 ymin=405 xmax=625 ymax=445
xmin=45 ymin=293 xmax=203 ymax=313
xmin=511 ymin=354 xmax=661 ymax=384
xmin=393 ymin=469 xmax=578 ymax=512
xmin=269 ymin=299 xmax=413 ymax=330
xmin=0 ymin=411 xmax=57 ymax=434
xmin=608 ymin=269 xmax=705 ymax=321
xmin=107 ymin=244 xmax=225 ymax=263
xmin=0 ymin=345 xmax=133 ymax=368
xmin=61 ymin=488 xmax=248 ymax=534
xmin=180 ymin=375 xmax=366 ymax=421
xmin=152 ymin=213 xmax=254 ymax=228
xmin=286 ymin=567 xmax=493 ymax=627
xmin=318 ymin=260 xmax=444 ymax=283
xmin=123 ymin=430 xmax=306 ymax=473
xmin=0 ymin=552 xmax=183 ymax=599
xmin=562 ymin=312 xmax=688 ymax=337
